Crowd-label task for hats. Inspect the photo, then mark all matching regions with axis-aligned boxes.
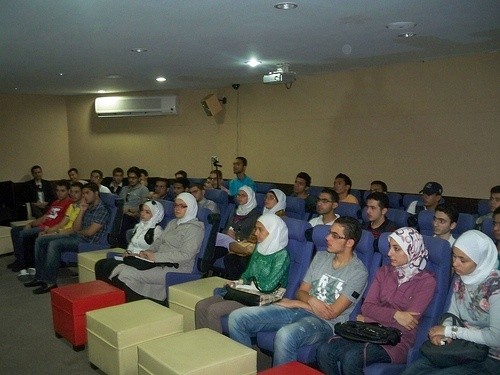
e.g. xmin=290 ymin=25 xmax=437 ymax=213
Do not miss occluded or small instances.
xmin=419 ymin=182 xmax=443 ymax=196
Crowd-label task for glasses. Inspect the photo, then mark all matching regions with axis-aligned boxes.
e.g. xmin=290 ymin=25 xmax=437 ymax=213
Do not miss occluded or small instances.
xmin=315 ymin=196 xmax=336 ymax=206
xmin=174 ymin=203 xmax=189 ymax=210
xmin=263 ymin=194 xmax=277 ymax=201
xmin=327 ymin=229 xmax=348 ymax=241
xmin=90 ymin=175 xmax=101 ymax=180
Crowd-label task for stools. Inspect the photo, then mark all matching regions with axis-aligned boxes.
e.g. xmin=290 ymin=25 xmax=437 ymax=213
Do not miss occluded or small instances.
xmin=0 ymin=219 xmax=36 ymax=255
xmin=257 ymin=361 xmax=324 ymax=375
xmin=50 ymin=280 xmax=126 ymax=352
xmin=138 ymin=328 xmax=257 ymax=375
xmin=169 ymin=276 xmax=229 ymax=331
xmin=86 ymin=298 xmax=183 ymax=375
xmin=78 ymin=247 xmax=126 ymax=283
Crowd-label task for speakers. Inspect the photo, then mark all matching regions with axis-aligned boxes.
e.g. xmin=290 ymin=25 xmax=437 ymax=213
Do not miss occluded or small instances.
xmin=200 ymin=94 xmax=223 ymax=117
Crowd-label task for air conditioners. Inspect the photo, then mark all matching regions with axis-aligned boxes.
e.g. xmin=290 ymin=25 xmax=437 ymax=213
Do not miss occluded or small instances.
xmin=95 ymin=95 xmax=181 ymax=118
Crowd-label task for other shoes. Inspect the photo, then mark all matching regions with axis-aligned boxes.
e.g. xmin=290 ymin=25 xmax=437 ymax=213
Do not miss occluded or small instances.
xmin=6 ymin=258 xmax=36 ymax=281
xmin=32 ymin=283 xmax=58 ymax=295
xmin=24 ymin=278 xmax=47 ymax=287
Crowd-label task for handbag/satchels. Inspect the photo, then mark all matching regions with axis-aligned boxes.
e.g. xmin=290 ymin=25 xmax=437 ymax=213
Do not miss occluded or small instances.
xmin=418 ymin=311 xmax=490 ymax=369
xmin=124 ymin=254 xmax=180 ymax=270
xmin=224 ymin=275 xmax=287 ymax=307
xmin=334 ymin=319 xmax=402 ymax=347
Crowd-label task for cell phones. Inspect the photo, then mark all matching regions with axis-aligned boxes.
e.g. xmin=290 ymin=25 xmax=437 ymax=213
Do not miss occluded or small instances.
xmin=439 ymin=338 xmax=449 ymax=346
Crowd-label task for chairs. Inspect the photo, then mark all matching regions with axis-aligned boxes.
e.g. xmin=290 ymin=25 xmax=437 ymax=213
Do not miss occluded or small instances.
xmin=60 ymin=171 xmax=494 ymax=375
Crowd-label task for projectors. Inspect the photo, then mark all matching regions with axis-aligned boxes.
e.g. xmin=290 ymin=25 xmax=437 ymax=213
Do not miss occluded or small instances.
xmin=263 ymin=67 xmax=297 ymax=87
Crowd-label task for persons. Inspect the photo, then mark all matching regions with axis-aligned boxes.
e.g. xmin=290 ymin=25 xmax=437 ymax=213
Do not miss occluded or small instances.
xmin=228 ymin=215 xmax=369 ymax=369
xmin=195 ymin=214 xmax=290 ymax=333
xmin=7 ymin=156 xmax=500 ymax=375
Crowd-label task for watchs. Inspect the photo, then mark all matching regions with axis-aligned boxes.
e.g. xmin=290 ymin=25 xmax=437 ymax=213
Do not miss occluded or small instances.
xmin=451 ymin=325 xmax=458 ymax=339
